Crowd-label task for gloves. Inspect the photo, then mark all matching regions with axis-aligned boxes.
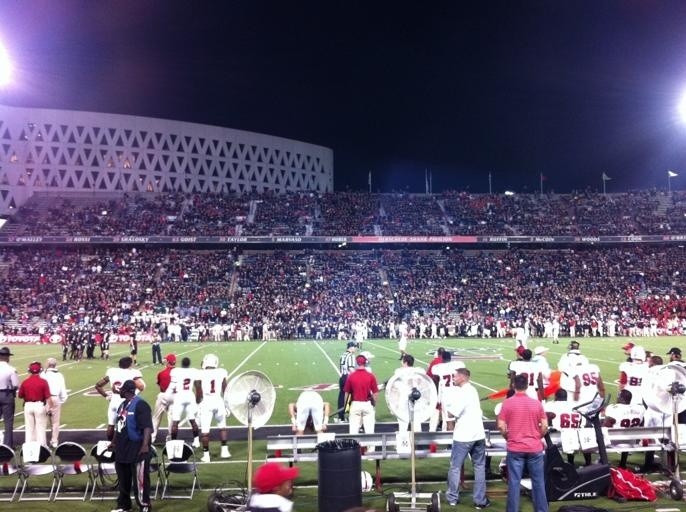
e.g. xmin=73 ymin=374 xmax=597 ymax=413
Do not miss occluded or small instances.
xmin=226 ymin=407 xmax=231 ymax=418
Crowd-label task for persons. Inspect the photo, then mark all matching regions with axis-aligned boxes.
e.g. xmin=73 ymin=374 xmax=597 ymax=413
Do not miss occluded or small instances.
xmin=0 ymin=185 xmax=685 ymax=512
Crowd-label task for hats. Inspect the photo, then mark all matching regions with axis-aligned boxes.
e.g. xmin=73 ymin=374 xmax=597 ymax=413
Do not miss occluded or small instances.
xmin=30 ymin=363 xmax=41 ymax=372
xmin=667 ymin=348 xmax=680 ymax=354
xmin=622 ymin=343 xmax=633 ymax=350
xmin=0 ymin=347 xmax=13 ymax=356
xmin=535 ymin=346 xmax=547 ymax=354
xmin=517 ymin=346 xmax=524 ymax=353
xmin=254 ymin=463 xmax=299 ymax=493
xmin=164 ymin=354 xmax=176 ymax=362
xmin=348 ymin=342 xmax=357 ymax=347
xmin=357 ymin=356 xmax=367 ymax=363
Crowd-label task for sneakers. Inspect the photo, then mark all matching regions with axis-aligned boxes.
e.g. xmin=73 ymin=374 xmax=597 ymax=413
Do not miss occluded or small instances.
xmin=221 ymin=451 xmax=232 ymax=459
xmin=201 ymin=456 xmax=210 ymax=462
xmin=475 ymin=498 xmax=490 ymax=510
xmin=192 ymin=442 xmax=201 ymax=448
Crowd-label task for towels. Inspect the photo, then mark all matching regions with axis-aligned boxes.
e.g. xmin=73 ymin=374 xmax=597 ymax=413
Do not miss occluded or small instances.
xmin=317 ymin=432 xmax=334 ymax=445
xmin=394 ymin=430 xmax=412 ymax=454
xmin=166 ymin=439 xmax=185 ymax=459
xmin=97 ymin=440 xmax=113 ymax=458
xmin=561 ymin=427 xmax=611 ymax=455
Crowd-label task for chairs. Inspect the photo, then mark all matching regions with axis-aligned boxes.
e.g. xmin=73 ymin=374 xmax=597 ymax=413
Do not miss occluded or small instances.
xmin=0 ymin=442 xmax=201 ymax=503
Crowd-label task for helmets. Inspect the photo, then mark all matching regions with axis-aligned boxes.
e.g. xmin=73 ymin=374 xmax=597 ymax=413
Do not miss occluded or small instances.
xmin=361 ymin=471 xmax=373 ymax=492
xmin=631 ymin=346 xmax=645 ymax=361
xmin=204 ymin=354 xmax=218 ymax=369
